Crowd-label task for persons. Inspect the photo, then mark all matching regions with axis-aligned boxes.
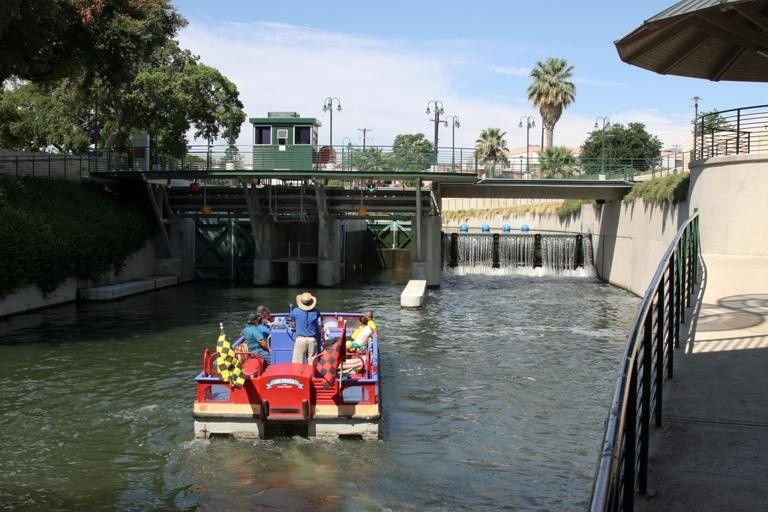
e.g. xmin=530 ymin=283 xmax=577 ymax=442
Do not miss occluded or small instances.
xmin=256 ymin=305 xmax=270 ymax=330
xmin=239 ymin=312 xmax=271 ymax=365
xmin=349 ymin=310 xmax=378 ymax=342
xmin=346 ymin=315 xmax=374 ymax=353
xmin=287 ymin=291 xmax=320 ymax=365
xmin=483 ymin=145 xmax=496 ymax=177
xmin=257 ymin=315 xmax=271 ymax=338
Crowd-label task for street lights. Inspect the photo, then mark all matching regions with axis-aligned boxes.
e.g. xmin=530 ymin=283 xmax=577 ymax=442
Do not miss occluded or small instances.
xmin=593 ymin=115 xmax=612 ymax=175
xmin=218 ymin=95 xmax=240 ymax=163
xmin=425 ymin=100 xmax=445 ymax=167
xmin=518 ymin=115 xmax=536 ymax=173
xmin=669 ymin=144 xmax=683 ymax=172
xmin=85 ymin=84 xmax=103 ymax=153
xmin=322 ymin=96 xmax=342 ymax=164
xmin=444 ymin=114 xmax=461 ymax=173
xmin=691 ymin=96 xmax=703 ymax=161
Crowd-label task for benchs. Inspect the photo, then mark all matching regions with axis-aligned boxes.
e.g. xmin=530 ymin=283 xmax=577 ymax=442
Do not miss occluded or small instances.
xmin=231 ymin=336 xmax=372 ymax=376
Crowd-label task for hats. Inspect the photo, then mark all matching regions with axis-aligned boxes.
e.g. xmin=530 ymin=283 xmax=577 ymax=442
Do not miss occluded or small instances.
xmin=247 ymin=314 xmax=261 ymax=323
xmin=296 ymin=293 xmax=317 ymax=311
xmin=258 ymin=304 xmax=265 ymax=312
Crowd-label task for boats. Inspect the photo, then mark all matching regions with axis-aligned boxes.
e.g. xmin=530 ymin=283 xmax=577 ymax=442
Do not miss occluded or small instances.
xmin=191 ymin=312 xmax=383 ymax=442
